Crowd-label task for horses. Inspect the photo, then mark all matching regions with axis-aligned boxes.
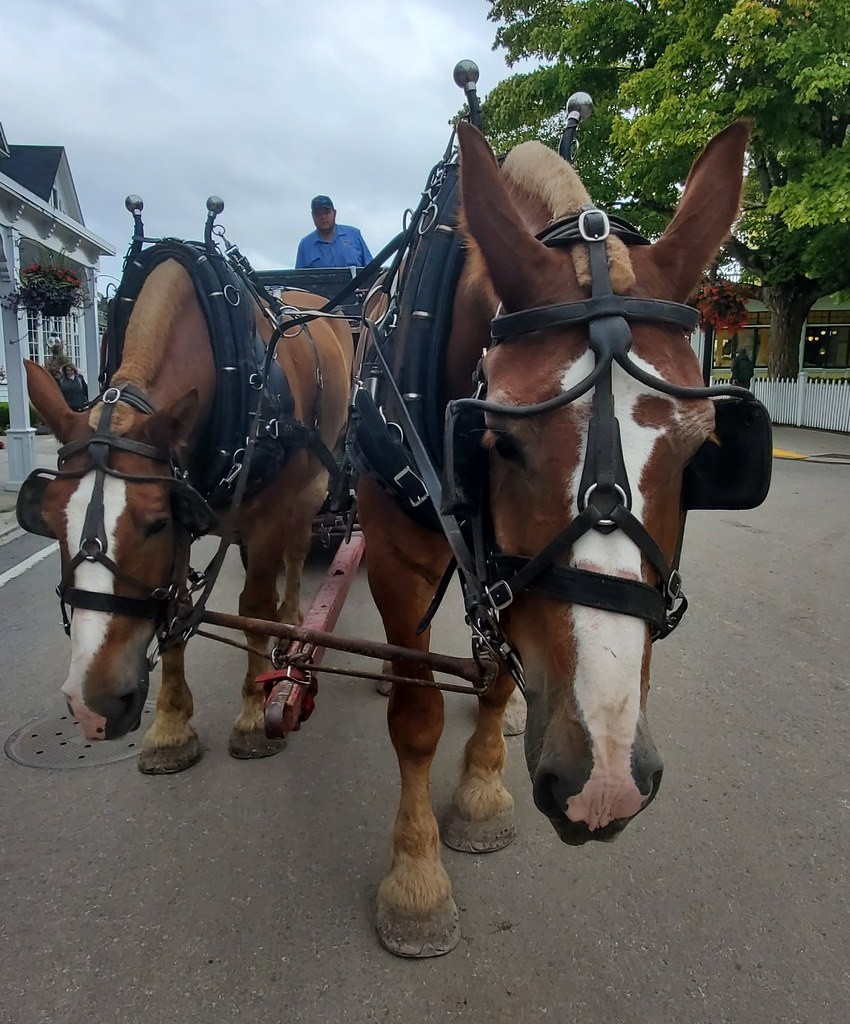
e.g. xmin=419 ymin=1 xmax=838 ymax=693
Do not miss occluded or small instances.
xmin=350 ymin=111 xmax=754 ymax=958
xmin=20 ymin=258 xmax=354 ymax=777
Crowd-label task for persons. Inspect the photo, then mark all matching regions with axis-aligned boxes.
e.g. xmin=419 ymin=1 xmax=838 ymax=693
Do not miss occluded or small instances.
xmin=294 ymin=195 xmax=374 ymax=269
xmin=729 ymin=347 xmax=754 ymax=390
xmin=60 ymin=363 xmax=89 ymax=411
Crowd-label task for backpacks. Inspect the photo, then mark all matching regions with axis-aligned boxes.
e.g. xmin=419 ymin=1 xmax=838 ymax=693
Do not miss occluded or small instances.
xmin=731 ymin=357 xmax=754 ymax=390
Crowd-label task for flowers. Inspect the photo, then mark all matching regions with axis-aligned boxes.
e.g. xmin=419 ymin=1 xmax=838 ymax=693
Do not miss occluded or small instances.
xmin=1 ymin=246 xmax=96 ymax=346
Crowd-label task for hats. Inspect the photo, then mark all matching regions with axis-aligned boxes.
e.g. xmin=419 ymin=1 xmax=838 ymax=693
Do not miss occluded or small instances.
xmin=310 ymin=194 xmax=335 ymax=211
xmin=734 ymin=348 xmax=746 ymax=355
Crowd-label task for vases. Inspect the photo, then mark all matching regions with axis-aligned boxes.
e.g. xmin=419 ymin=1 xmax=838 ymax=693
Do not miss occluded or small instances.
xmin=42 ymin=300 xmax=72 ymax=317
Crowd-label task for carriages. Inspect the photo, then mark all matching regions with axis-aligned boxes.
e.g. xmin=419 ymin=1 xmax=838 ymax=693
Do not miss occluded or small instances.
xmin=17 ymin=119 xmax=772 ymax=958
